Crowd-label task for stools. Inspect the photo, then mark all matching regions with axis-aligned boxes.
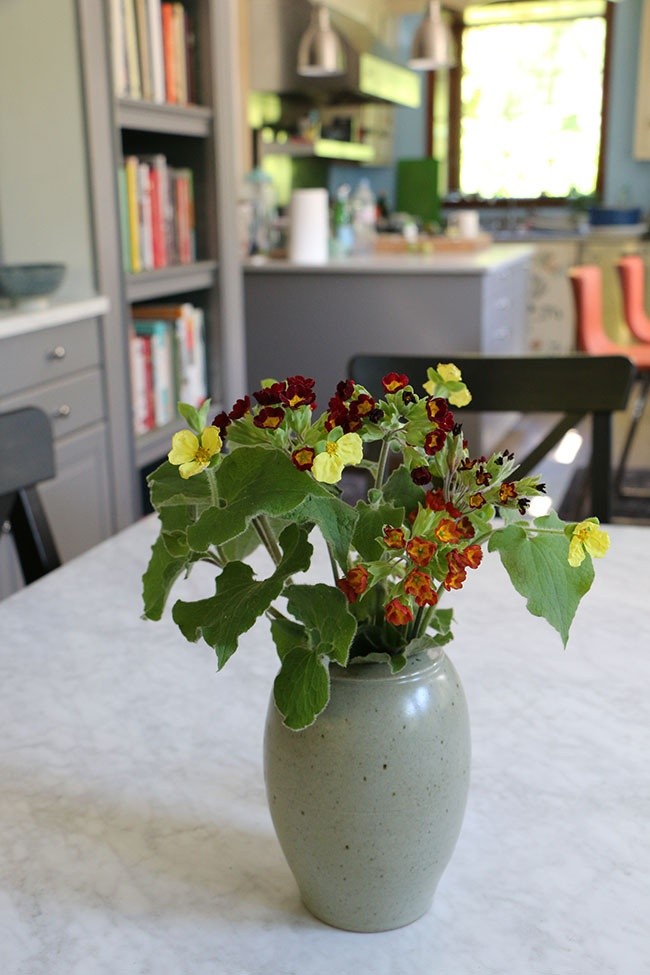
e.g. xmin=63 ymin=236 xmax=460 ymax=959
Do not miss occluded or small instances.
xmin=570 ymin=258 xmax=650 ymax=498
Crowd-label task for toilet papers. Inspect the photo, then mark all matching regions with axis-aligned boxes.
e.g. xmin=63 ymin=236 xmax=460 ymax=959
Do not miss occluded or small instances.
xmin=287 ymin=186 xmax=330 ymax=265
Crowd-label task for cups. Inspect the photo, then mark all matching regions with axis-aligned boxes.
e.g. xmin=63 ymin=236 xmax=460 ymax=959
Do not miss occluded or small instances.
xmin=447 ymin=209 xmax=476 ymax=240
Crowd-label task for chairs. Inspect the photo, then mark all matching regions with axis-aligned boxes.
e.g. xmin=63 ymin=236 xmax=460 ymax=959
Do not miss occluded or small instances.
xmin=350 ymin=352 xmax=633 ymax=525
xmin=0 ymin=406 xmax=64 ymax=585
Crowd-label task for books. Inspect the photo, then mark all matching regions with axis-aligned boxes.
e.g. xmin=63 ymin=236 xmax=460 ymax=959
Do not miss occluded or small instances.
xmin=129 ymin=302 xmax=209 ymax=436
xmin=126 ymin=153 xmax=197 ymax=272
xmin=121 ymin=0 xmax=189 ymax=105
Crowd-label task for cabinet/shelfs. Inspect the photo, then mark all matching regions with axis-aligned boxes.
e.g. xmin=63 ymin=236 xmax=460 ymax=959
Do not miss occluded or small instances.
xmin=0 ymin=299 xmax=116 ymax=602
xmin=77 ymin=0 xmax=247 ymax=530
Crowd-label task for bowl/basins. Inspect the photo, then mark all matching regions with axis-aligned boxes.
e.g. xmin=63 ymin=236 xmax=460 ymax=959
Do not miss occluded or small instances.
xmin=0 ymin=263 xmax=64 ymax=296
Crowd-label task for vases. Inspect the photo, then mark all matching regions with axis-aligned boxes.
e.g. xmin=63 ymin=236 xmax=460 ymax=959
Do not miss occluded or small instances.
xmin=261 ymin=635 xmax=470 ymax=933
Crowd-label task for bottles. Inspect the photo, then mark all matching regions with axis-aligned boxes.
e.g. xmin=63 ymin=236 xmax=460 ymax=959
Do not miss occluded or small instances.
xmin=353 ymin=179 xmax=377 ymax=239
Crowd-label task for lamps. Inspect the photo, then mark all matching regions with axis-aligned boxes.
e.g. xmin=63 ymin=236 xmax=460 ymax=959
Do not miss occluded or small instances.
xmin=407 ymin=0 xmax=456 ymax=70
xmin=295 ymin=0 xmax=346 ymax=77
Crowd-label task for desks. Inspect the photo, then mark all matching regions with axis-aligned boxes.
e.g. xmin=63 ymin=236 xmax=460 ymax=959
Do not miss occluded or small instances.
xmin=0 ymin=513 xmax=650 ymax=974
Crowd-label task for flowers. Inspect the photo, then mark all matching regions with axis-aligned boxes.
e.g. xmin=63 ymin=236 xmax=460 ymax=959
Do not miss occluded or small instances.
xmin=143 ymin=363 xmax=609 ymax=730
